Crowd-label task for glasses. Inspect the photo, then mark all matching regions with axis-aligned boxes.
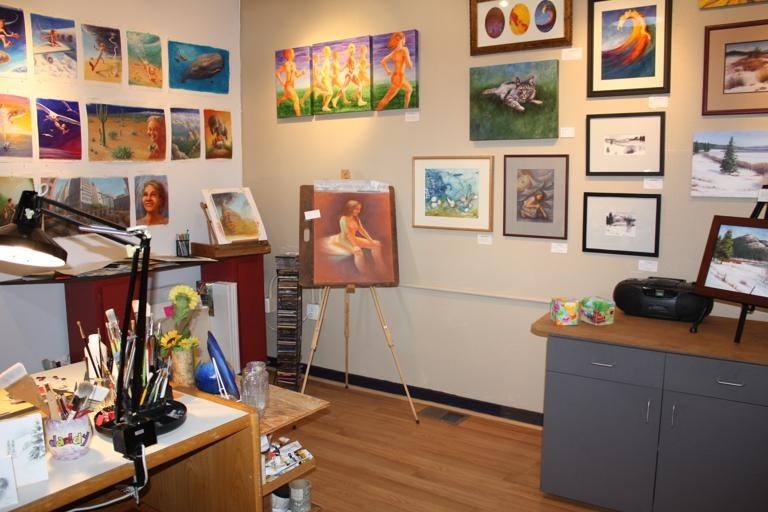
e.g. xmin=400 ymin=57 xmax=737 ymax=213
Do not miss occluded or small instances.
xmin=0 ymin=191 xmax=186 ymax=437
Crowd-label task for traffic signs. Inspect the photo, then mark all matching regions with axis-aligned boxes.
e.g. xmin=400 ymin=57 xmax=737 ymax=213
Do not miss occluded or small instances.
xmin=306 ymin=304 xmax=320 ymax=320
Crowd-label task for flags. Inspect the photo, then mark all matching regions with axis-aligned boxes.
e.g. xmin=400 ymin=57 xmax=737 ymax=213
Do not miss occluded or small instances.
xmin=173 ymin=351 xmax=194 ymax=386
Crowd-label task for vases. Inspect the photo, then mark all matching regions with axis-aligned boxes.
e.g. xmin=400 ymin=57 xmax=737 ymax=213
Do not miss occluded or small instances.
xmin=154 ymin=285 xmax=201 ymax=355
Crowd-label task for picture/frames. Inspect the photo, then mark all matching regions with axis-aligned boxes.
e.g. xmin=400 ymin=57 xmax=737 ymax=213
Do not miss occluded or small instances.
xmin=528 ymin=306 xmax=768 ymax=512
xmin=0 ymin=361 xmax=264 ymax=512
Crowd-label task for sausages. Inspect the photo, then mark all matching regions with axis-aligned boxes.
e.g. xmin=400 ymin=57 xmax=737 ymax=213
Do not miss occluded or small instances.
xmin=483 ymin=72 xmax=544 ymax=113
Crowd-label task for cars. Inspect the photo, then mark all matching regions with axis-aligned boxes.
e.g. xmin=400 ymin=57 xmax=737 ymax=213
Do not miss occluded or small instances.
xmin=289 ymin=480 xmax=311 ymax=512
xmin=43 ymin=415 xmax=95 ymax=462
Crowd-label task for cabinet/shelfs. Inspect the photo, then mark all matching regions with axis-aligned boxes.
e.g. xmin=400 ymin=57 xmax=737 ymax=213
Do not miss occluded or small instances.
xmin=240 ymin=361 xmax=270 ymax=418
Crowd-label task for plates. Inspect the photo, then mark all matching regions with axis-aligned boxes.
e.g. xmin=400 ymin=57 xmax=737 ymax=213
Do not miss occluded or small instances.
xmin=201 ymin=254 xmax=271 ymax=369
xmin=66 ymin=276 xmax=142 ymax=363
xmin=214 ymin=374 xmax=330 ymax=512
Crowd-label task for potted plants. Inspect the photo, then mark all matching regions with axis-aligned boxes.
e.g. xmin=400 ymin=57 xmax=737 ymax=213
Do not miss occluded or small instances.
xmin=585 ymin=110 xmax=666 ymax=178
xmin=202 ymin=187 xmax=268 ymax=244
xmin=701 ymin=18 xmax=768 ymax=117
xmin=412 ymin=155 xmax=494 ymax=233
xmin=580 ymin=192 xmax=662 ymax=259
xmin=469 ymin=0 xmax=575 ymax=56
xmin=694 ymin=216 xmax=768 ymax=307
xmin=587 ymin=0 xmax=672 ymax=96
xmin=502 ymin=153 xmax=570 ymax=241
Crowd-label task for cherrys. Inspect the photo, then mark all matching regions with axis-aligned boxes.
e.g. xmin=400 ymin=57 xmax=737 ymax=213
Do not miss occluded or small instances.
xmin=0 ymin=363 xmax=51 ymax=418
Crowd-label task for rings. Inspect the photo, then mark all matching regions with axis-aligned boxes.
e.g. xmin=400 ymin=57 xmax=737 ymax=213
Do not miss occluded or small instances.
xmin=175 ymin=229 xmax=189 ymax=257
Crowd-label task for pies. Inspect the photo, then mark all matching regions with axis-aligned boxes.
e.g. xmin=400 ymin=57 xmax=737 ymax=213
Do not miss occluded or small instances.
xmin=154 ymin=370 xmax=167 ymax=402
xmin=149 ymin=369 xmax=164 ymax=402
xmin=61 ymin=399 xmax=70 ymax=416
xmin=146 ymin=302 xmax=151 ymax=340
xmin=56 ymin=398 xmax=69 ymax=421
xmin=105 ymin=308 xmax=118 ymax=327
xmin=155 ymin=322 xmax=161 ymax=345
xmin=72 ymin=400 xmax=85 ymax=420
xmin=140 ymin=370 xmax=153 ymax=405
xmin=131 ymin=319 xmax=135 ymax=336
xmin=43 ymin=384 xmax=61 ymax=423
xmin=120 ymin=336 xmax=156 ymax=392
xmin=77 ymin=320 xmax=101 ymax=378
xmin=105 ymin=322 xmax=121 ymax=355
xmin=131 ymin=299 xmax=140 ymax=321
